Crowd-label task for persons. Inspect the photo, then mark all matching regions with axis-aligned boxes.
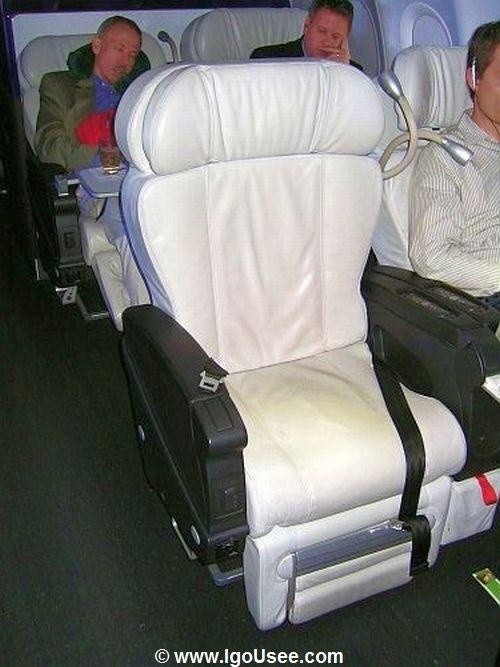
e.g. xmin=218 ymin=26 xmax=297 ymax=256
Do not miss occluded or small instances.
xmin=409 ymin=20 xmax=500 ymax=342
xmin=250 ymin=0 xmax=363 ymax=72
xmin=34 ymin=16 xmax=151 ymax=306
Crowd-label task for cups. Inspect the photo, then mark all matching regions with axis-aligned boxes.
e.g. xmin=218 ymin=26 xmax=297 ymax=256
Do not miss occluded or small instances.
xmin=97 ymin=136 xmax=120 ymax=174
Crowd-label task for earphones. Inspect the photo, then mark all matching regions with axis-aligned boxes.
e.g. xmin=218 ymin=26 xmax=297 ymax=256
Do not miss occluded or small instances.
xmin=471 ymin=57 xmax=479 ymax=90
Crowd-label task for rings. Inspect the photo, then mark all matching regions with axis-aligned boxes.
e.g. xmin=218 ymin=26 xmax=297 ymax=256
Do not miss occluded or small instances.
xmin=336 ymin=53 xmax=340 ymax=57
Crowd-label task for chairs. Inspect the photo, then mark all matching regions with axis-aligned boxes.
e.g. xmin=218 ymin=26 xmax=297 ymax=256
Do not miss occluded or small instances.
xmin=15 ymin=5 xmax=498 ymax=636
